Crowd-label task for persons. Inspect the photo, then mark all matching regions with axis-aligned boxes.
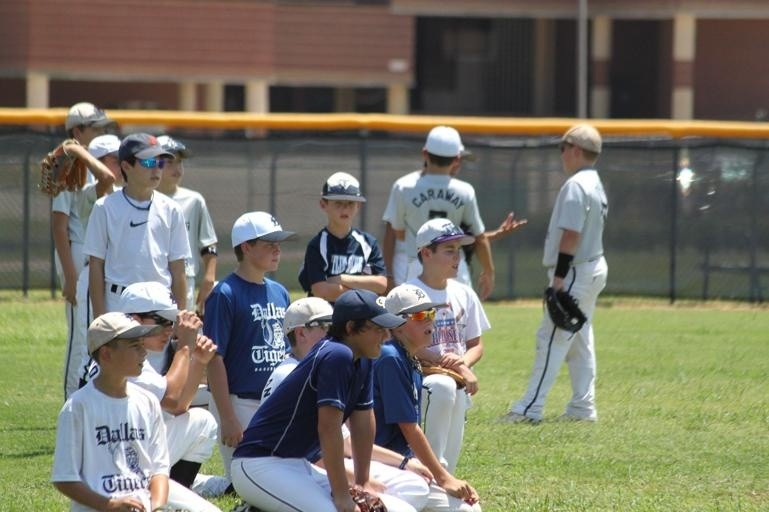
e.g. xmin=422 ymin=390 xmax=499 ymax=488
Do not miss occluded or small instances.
xmin=406 ymin=218 xmax=490 ymax=478
xmin=77 ymin=281 xmax=235 ymax=498
xmin=230 ymin=289 xmax=417 ymax=512
xmin=492 ymin=124 xmax=608 ymax=426
xmin=40 ymin=104 xmax=123 ymax=402
xmin=382 ymin=126 xmax=526 ymax=301
xmin=83 ymin=132 xmax=191 ymax=375
xmin=372 ymin=282 xmax=479 ymax=510
xmin=201 ymin=211 xmax=299 ymax=471
xmin=49 ymin=312 xmax=218 ymax=510
xmin=297 ymin=171 xmax=388 ymax=315
xmin=154 ymin=134 xmax=219 ymax=313
xmin=262 ymin=297 xmax=434 ymax=510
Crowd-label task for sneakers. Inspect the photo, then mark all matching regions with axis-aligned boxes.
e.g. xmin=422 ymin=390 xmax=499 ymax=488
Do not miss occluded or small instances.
xmin=488 ymin=409 xmax=541 ymax=428
xmin=550 ymin=410 xmax=599 ymax=425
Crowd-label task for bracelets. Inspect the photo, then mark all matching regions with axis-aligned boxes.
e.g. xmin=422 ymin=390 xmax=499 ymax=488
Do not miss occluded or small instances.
xmin=399 ymin=457 xmax=408 ymax=469
xmin=386 ymin=273 xmax=392 ymax=277
xmin=554 ymin=252 xmax=574 ymax=279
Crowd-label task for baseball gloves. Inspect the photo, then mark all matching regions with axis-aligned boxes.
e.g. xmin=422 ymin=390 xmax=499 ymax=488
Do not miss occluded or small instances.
xmin=37 ymin=138 xmax=88 ymax=197
xmin=545 ymin=288 xmax=586 ymax=333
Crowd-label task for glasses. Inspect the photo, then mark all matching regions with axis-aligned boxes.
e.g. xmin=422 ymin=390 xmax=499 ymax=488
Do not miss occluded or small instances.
xmin=402 ymin=310 xmax=437 ymax=322
xmin=142 ymin=314 xmax=175 ymax=326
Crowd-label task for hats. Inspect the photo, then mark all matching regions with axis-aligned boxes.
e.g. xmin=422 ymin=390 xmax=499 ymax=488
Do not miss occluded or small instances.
xmin=414 ymin=216 xmax=476 ymax=254
xmin=231 ymin=210 xmax=297 ymax=246
xmin=118 ymin=282 xmax=183 ymax=322
xmin=550 ymin=125 xmax=602 ymax=154
xmin=424 ymin=126 xmax=473 ymax=160
xmin=87 ymin=134 xmax=122 ymax=160
xmin=64 ymin=101 xmax=118 ymax=132
xmin=118 ymin=133 xmax=176 ymax=163
xmin=320 ymin=170 xmax=367 ymax=204
xmin=384 ymin=283 xmax=449 ymax=317
xmin=87 ymin=310 xmax=163 ymax=357
xmin=156 ymin=134 xmax=186 ymax=157
xmin=284 ymin=296 xmax=335 ymax=336
xmin=332 ymin=288 xmax=407 ymax=331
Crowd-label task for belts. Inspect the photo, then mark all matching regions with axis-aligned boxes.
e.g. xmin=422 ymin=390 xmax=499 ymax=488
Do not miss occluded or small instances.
xmin=107 ymin=281 xmax=127 ymax=298
xmin=228 ymin=389 xmax=263 ymax=402
